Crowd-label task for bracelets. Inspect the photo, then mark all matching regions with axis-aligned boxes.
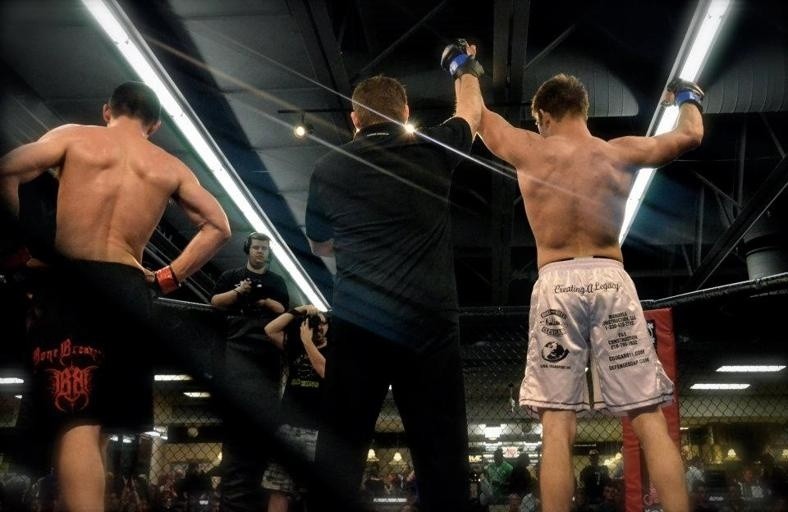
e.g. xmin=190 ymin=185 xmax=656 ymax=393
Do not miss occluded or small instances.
xmin=153 ymin=262 xmax=182 ymax=298
xmin=1 ymin=247 xmax=33 ymax=272
xmin=233 ymin=289 xmax=243 ymax=296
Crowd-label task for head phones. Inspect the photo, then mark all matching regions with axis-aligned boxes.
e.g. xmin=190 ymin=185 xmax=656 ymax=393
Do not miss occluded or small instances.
xmin=242 ymin=232 xmax=272 ymax=260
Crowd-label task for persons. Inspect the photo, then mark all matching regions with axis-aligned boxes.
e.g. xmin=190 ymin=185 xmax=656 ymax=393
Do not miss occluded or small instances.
xmin=438 ymin=40 xmax=706 ymax=512
xmin=208 ymin=231 xmax=290 ymax=490
xmin=263 ymin=302 xmax=330 ymax=512
xmin=1 ymin=449 xmax=786 ymax=510
xmin=1 ymin=78 xmax=232 ymax=510
xmin=304 ymin=36 xmax=488 ymax=510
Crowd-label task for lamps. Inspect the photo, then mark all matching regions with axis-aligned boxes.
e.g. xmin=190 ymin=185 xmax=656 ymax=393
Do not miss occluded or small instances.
xmin=619 ymin=0 xmax=734 ymax=250
xmin=77 ymin=0 xmax=333 ymax=314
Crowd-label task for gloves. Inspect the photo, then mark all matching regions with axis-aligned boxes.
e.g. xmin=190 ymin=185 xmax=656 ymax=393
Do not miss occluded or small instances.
xmin=666 ymin=79 xmax=705 ymax=112
xmin=441 ymin=39 xmax=485 ymax=80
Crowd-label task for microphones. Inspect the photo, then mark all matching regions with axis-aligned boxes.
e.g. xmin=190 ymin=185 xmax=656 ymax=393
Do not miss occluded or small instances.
xmin=267 ymin=258 xmax=271 ymax=275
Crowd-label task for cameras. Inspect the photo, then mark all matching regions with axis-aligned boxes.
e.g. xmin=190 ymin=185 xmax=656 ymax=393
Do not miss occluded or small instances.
xmin=305 ymin=314 xmax=321 ymax=327
